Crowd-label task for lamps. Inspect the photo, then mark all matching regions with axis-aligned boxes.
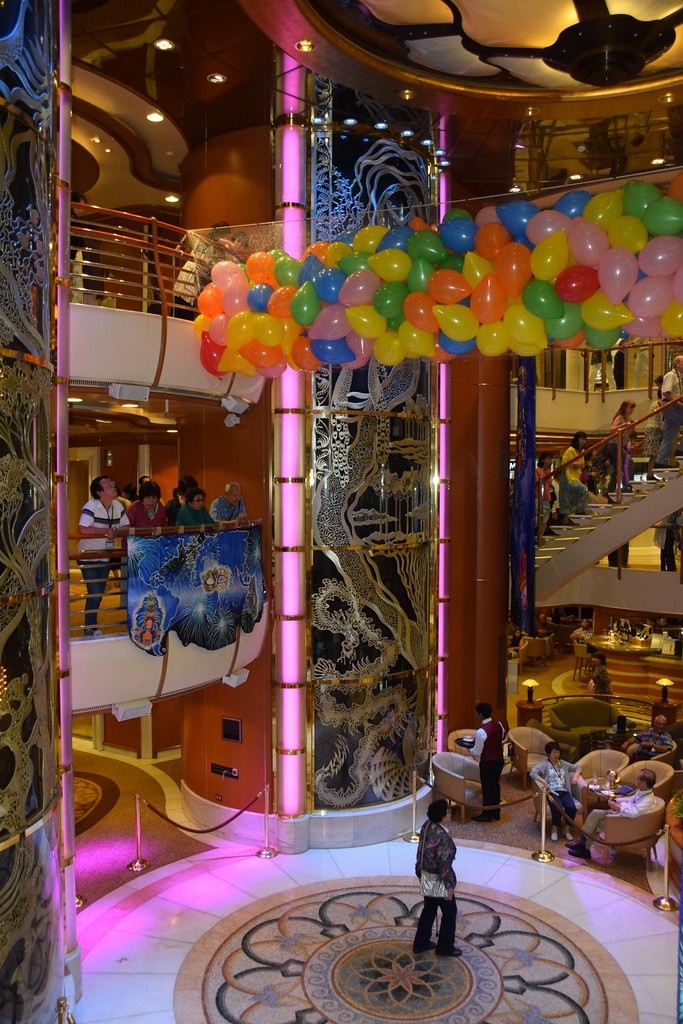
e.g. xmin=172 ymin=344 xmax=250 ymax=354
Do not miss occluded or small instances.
xmin=656 ymin=678 xmax=674 ymax=703
xmin=522 ymin=679 xmax=540 ymax=703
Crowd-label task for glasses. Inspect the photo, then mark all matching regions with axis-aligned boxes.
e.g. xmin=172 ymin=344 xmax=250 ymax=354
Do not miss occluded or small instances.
xmin=193 ymin=498 xmax=204 ymax=503
xmin=102 ymin=481 xmax=115 ymax=489
xmin=446 ymin=807 xmax=452 ymax=813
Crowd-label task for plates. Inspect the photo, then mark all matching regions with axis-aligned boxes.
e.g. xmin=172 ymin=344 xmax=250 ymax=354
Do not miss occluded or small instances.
xmin=463 ymin=738 xmax=473 ymax=742
xmin=588 ymin=784 xmax=600 ymax=790
xmin=601 ymin=791 xmax=614 ymax=794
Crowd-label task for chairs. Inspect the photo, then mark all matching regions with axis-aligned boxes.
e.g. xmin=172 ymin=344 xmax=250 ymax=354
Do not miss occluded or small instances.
xmin=432 ymin=697 xmax=683 ymax=871
xmin=572 ymin=639 xmax=592 ymax=682
xmin=507 ymin=621 xmax=576 ymax=676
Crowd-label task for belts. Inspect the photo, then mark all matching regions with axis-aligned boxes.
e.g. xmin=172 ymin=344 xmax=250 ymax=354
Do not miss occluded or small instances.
xmin=662 ymin=398 xmax=669 ymax=402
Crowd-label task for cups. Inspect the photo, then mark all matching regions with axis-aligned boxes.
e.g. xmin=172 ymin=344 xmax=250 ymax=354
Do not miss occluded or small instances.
xmin=463 ymin=736 xmax=471 ymax=741
xmin=593 ymin=774 xmax=597 ymax=785
xmin=606 ymin=777 xmax=610 ymax=790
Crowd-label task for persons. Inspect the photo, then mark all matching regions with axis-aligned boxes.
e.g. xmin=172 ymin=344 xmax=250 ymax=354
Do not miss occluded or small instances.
xmin=608 ymin=541 xmax=631 ymax=567
xmin=529 ymin=741 xmax=582 ymax=841
xmin=592 ymin=665 xmax=615 ymax=704
xmin=607 ymin=399 xmax=637 ymax=493
xmin=76 ymin=475 xmax=169 ymax=635
xmin=69 ymin=191 xmax=102 ymax=305
xmin=413 ymin=799 xmax=462 ymax=957
xmin=173 ymin=221 xmax=238 ymax=321
xmin=165 ymin=478 xmax=248 ymax=526
xmin=613 ymin=619 xmax=629 ymax=630
xmin=507 ymin=614 xmax=565 ymax=659
xmin=652 ymin=508 xmax=683 ymax=572
xmin=536 ymin=431 xmax=609 ymax=547
xmin=621 ymin=715 xmax=673 ymax=766
xmin=214 ymin=230 xmax=249 ymax=264
xmin=564 ymin=768 xmax=657 ymax=859
xmin=613 ymin=340 xmax=625 ymax=390
xmin=646 ymin=355 xmax=683 ymax=482
xmin=467 ymin=703 xmax=506 ymax=822
xmin=569 ymin=619 xmax=606 ymax=670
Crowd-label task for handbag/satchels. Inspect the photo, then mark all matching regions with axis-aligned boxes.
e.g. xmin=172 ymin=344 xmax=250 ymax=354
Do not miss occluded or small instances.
xmin=579 ymin=466 xmax=589 ymax=482
xmin=420 ymin=871 xmax=448 ymax=898
xmin=587 ymin=679 xmax=596 ymax=695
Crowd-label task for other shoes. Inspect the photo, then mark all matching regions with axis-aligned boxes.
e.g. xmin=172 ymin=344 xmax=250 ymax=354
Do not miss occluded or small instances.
xmin=568 ymin=848 xmax=591 ymax=858
xmin=436 ymin=948 xmax=462 ymax=957
xmin=413 ymin=941 xmax=437 ymax=953
xmin=492 ymin=813 xmax=500 ymax=820
xmin=541 ymin=529 xmax=560 ymax=539
xmin=472 ymin=814 xmax=491 ymax=822
xmin=550 ymin=830 xmax=558 ymax=841
xmin=574 ymin=508 xmax=596 ymax=515
xmin=654 ymin=459 xmax=681 ymax=469
xmin=559 ymin=518 xmax=581 ymax=526
xmin=565 ymin=839 xmax=586 ymax=851
xmin=562 ymin=830 xmax=574 ymax=840
xmin=621 ymin=485 xmax=639 ymax=493
xmin=646 ymin=473 xmax=664 ymax=482
xmin=84 ymin=627 xmax=102 ymax=635
xmin=603 ymin=492 xmax=617 ymax=504
xmin=115 ymin=581 xmax=121 ymax=588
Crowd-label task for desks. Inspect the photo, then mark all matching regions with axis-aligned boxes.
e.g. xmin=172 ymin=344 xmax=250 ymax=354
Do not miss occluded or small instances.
xmin=582 ymin=778 xmax=638 ymax=826
xmin=589 ymin=634 xmax=662 ymax=657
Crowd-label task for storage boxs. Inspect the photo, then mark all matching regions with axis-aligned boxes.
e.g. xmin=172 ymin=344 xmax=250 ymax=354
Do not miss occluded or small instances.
xmin=632 ymin=624 xmax=653 ymax=647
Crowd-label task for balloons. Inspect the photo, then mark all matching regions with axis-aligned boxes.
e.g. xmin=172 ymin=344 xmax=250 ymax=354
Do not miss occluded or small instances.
xmin=195 ymin=216 xmax=479 ymax=379
xmin=461 ymin=173 xmax=683 ymax=355
